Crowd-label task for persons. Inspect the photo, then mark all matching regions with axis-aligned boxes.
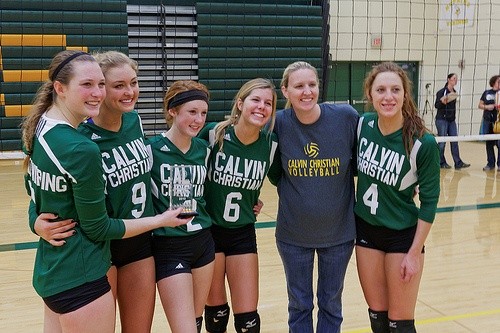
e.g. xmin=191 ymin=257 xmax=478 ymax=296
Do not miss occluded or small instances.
xmin=197 ymin=78 xmax=282 ymax=333
xmin=478 ymin=75 xmax=500 ymax=171
xmin=28 ymin=51 xmax=156 ymax=333
xmin=22 ymin=50 xmax=193 ymax=333
xmin=354 ymin=62 xmax=441 ymax=333
xmin=144 ymin=80 xmax=264 ymax=333
xmin=435 ymin=73 xmax=471 ymax=169
xmin=265 ymin=61 xmax=420 ymax=333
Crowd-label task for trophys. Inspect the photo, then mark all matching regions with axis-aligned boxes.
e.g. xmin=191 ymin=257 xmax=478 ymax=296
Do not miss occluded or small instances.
xmin=170 ymin=166 xmax=199 ymax=216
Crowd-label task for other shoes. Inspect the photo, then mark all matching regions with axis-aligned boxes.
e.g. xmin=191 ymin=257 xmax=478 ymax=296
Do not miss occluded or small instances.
xmin=497 ymin=165 xmax=500 ymax=171
xmin=440 ymin=163 xmax=452 ymax=168
xmin=455 ymin=162 xmax=471 ymax=169
xmin=483 ymin=164 xmax=496 ymax=169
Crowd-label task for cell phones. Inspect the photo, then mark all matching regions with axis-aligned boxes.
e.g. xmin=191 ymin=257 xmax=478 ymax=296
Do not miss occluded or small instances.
xmin=176 ymin=212 xmax=199 ymax=219
